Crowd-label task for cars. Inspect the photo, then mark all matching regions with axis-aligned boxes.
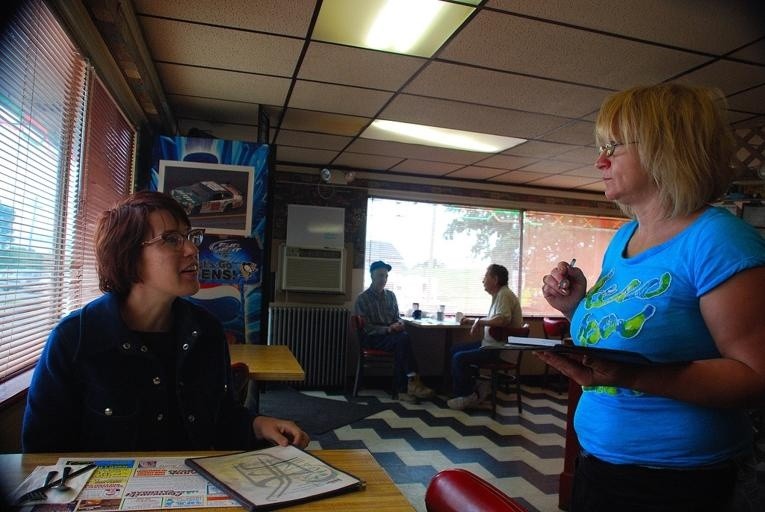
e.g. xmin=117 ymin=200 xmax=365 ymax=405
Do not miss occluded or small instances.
xmin=0 ymin=267 xmax=51 ymax=291
xmin=170 ymin=181 xmax=245 ymax=216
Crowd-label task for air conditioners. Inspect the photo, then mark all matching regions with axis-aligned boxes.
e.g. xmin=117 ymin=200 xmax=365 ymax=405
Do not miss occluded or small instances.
xmin=282 ymin=243 xmax=348 ymax=295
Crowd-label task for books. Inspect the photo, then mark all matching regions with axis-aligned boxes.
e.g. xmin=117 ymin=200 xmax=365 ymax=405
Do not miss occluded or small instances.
xmin=502 ymin=335 xmax=645 ymax=364
xmin=185 ymin=442 xmax=363 ymax=512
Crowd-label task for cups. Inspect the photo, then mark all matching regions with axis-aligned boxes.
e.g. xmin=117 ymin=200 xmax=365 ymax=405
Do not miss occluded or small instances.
xmin=412 ymin=302 xmax=463 ymax=322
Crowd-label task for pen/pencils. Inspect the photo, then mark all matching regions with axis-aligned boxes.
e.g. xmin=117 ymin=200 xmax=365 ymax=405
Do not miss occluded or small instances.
xmin=558 ymin=259 xmax=576 ymax=290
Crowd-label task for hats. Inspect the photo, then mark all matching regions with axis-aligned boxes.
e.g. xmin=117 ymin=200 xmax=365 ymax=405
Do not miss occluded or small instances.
xmin=370 ymin=261 xmax=392 ymax=271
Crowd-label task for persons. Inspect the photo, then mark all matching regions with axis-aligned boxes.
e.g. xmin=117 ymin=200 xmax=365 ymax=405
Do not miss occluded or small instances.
xmin=531 ymin=80 xmax=765 ymax=512
xmin=354 ymin=259 xmax=435 ymax=405
xmin=444 ymin=264 xmax=524 ymax=410
xmin=21 ymin=189 xmax=311 ymax=452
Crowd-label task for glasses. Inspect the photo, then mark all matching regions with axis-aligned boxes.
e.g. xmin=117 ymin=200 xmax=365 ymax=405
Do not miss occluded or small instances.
xmin=139 ymin=228 xmax=205 ymax=252
xmin=598 ymin=139 xmax=638 ymax=157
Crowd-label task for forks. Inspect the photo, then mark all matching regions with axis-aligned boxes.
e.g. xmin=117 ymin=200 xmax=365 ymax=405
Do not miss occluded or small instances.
xmin=27 ymin=471 xmax=58 ymax=500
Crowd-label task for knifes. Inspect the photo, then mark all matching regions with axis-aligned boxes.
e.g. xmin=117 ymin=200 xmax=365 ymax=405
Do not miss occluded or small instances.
xmin=15 ymin=464 xmax=97 ymax=504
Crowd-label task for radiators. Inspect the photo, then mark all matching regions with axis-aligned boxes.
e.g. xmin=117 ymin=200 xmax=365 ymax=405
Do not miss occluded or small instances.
xmin=266 ymin=302 xmax=348 ymax=395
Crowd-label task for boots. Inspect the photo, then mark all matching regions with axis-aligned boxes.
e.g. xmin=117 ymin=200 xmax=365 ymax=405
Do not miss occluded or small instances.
xmin=408 ymin=376 xmax=433 ymax=399
xmin=399 ymin=393 xmax=419 ymax=405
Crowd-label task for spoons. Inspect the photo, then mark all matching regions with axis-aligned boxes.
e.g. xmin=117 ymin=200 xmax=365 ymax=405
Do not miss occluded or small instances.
xmin=51 ymin=467 xmax=71 ymax=492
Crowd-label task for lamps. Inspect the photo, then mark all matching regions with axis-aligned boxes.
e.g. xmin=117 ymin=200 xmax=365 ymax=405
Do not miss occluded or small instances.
xmin=320 ymin=165 xmax=356 ymax=184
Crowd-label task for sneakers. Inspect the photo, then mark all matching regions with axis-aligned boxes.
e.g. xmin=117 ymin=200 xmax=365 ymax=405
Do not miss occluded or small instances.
xmin=447 ymin=392 xmax=478 ymax=410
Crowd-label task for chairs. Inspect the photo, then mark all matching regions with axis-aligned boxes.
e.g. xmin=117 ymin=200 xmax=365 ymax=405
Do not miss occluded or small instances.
xmin=543 ymin=318 xmax=570 ymax=394
xmin=474 ymin=324 xmax=529 ymax=416
xmin=350 ymin=315 xmax=400 ymax=400
xmin=230 ymin=363 xmax=259 ymax=412
xmin=226 ymin=331 xmax=242 ymax=345
xmin=424 ymin=468 xmax=527 ymax=512
